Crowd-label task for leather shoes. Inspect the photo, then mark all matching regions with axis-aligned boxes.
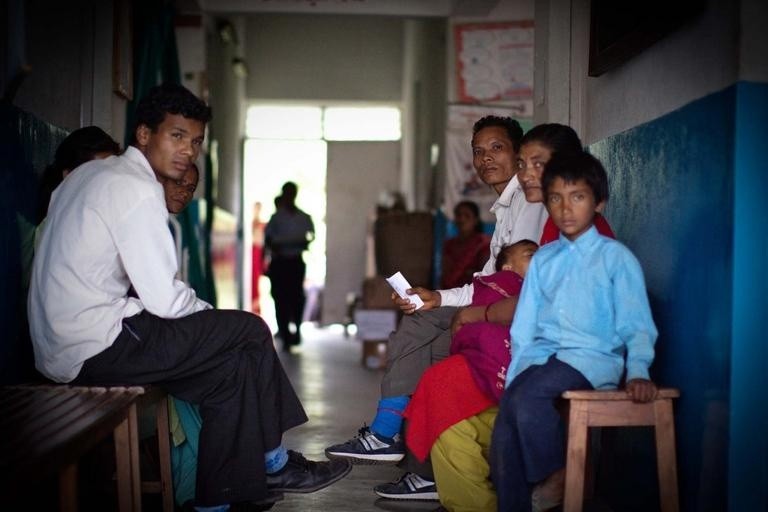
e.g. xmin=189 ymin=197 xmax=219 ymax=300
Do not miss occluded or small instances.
xmin=229 ymin=449 xmax=353 ymax=510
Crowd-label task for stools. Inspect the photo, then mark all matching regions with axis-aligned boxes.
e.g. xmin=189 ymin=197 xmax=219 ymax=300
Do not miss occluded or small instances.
xmin=1 ymin=384 xmax=173 ymax=506
xmin=564 ymin=387 xmax=685 ymax=511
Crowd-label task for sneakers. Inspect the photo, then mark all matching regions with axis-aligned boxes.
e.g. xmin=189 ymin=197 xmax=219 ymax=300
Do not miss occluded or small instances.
xmin=324 ymin=428 xmax=405 ymax=465
xmin=373 ymin=472 xmax=441 ymax=502
